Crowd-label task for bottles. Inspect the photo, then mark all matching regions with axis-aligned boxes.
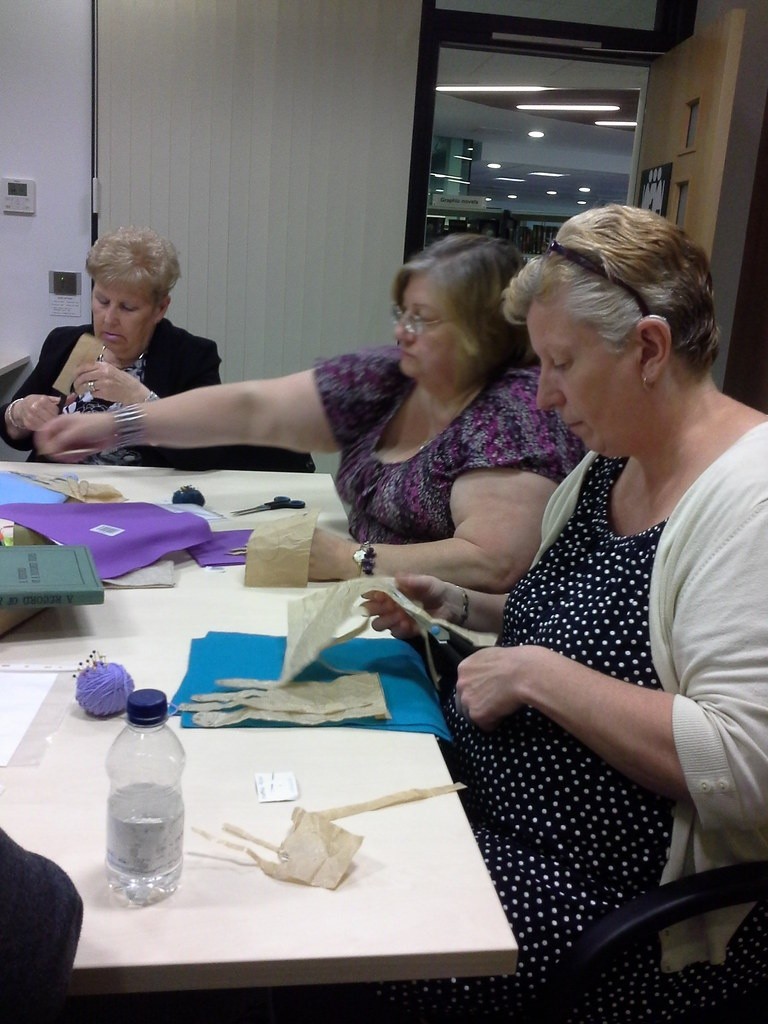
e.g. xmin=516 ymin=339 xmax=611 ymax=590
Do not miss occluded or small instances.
xmin=106 ymin=688 xmax=186 ymax=908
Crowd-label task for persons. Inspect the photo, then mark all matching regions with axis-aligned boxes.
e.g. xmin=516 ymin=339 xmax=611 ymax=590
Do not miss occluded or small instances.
xmin=0 ymin=225 xmax=222 ymax=472
xmin=363 ymin=202 xmax=768 ymax=1024
xmin=34 ymin=231 xmax=589 ymax=595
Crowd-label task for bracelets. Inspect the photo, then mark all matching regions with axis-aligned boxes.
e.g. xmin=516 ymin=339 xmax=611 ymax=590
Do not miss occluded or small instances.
xmin=112 ymin=398 xmax=145 ymax=449
xmin=9 ymin=398 xmax=26 ymax=429
xmin=145 ymin=392 xmax=159 ymax=402
xmin=454 ymin=587 xmax=469 ymax=625
xmin=354 ymin=539 xmax=376 ymax=577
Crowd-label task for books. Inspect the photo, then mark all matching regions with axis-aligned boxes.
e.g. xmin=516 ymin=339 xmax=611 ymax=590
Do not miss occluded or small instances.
xmin=0 ymin=545 xmax=104 ymax=609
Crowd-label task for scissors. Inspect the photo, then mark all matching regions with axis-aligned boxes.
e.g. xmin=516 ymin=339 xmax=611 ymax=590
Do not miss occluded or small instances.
xmin=390 ymin=585 xmax=530 ymax=737
xmin=55 ymin=344 xmax=109 ymax=417
xmin=231 ymin=494 xmax=308 ymax=519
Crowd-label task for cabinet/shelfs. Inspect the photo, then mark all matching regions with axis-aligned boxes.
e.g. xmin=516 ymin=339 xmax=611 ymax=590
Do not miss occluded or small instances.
xmin=426 ymin=204 xmax=575 ymax=260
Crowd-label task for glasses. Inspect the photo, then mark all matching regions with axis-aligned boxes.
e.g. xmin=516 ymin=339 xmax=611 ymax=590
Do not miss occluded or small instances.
xmin=545 ymin=237 xmax=650 ymax=319
xmin=391 ymin=303 xmax=458 ymax=336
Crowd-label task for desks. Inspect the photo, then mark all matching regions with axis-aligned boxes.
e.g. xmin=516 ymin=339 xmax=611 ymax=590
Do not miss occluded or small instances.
xmin=1 ymin=461 xmax=519 ymax=997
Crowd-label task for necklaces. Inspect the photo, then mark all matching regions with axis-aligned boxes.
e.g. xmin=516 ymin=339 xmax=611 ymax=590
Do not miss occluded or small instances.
xmin=99 ymin=345 xmax=144 ymax=372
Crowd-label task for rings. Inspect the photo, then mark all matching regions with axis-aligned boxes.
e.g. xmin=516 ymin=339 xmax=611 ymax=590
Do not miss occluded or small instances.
xmin=90 ymin=381 xmax=95 ymax=391
